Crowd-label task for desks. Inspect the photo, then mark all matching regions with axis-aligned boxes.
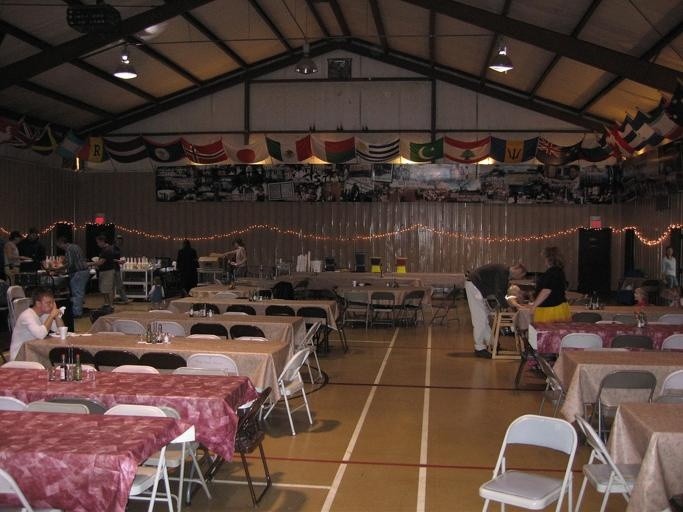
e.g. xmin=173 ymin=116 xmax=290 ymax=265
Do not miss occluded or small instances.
xmin=0 ymin=271 xmax=683 ymax=512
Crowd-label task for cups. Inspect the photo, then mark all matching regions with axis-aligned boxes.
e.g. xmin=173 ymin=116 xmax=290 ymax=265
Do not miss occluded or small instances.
xmin=59 ymin=326 xmax=68 ymax=340
xmin=352 ymin=281 xmax=356 ymax=287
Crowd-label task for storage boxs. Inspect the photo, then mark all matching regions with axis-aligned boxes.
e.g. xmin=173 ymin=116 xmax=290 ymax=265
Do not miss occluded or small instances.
xmin=198 ymin=256 xmax=222 ymax=268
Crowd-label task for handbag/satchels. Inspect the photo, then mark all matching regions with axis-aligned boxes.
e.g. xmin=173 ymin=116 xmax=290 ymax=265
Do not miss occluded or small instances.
xmin=232 ymin=411 xmax=264 ymax=452
xmin=514 ymin=308 xmax=532 ymax=330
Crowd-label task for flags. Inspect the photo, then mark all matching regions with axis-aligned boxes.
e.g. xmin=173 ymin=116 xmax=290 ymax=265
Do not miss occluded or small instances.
xmin=2 ymin=116 xmax=609 ymax=165
xmin=596 ymin=78 xmax=682 ymax=162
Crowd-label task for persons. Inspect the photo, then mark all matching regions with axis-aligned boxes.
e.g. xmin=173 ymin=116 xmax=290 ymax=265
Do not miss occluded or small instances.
xmin=505 ymin=284 xmax=526 ymax=317
xmin=464 ymin=263 xmax=526 ymax=357
xmin=3 ymin=228 xmax=247 ymax=371
xmin=660 ymin=246 xmax=679 ymax=288
xmin=526 ymin=247 xmax=571 ymax=370
xmin=633 ymin=287 xmax=648 ymax=328
xmin=239 ymin=166 xmax=582 ymax=201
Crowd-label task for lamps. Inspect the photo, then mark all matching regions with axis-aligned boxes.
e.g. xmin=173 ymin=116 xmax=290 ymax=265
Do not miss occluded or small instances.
xmin=487 ymin=43 xmax=515 ymax=73
xmin=113 ymin=45 xmax=137 ymax=79
xmin=293 ymin=43 xmax=320 ymax=74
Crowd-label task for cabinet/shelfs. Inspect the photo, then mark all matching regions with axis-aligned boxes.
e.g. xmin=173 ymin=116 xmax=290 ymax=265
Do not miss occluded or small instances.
xmin=197 ymin=268 xmax=224 ymax=286
xmin=115 ymin=267 xmax=154 ymax=302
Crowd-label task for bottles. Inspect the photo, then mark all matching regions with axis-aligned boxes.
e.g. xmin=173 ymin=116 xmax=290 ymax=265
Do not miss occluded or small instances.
xmin=209 ymin=309 xmax=212 ymax=317
xmin=74 ymin=355 xmax=82 ymax=381
xmin=146 ymin=322 xmax=169 ymax=344
xmin=60 ymin=355 xmax=67 ymax=382
xmin=249 ymin=290 xmax=253 ymax=302
xmin=119 ymin=256 xmax=149 ymax=271
xmin=253 ymin=288 xmax=257 ymax=301
xmin=189 ymin=302 xmax=194 ymax=317
xmin=46 ymin=256 xmax=59 ymax=268
xmin=202 ymin=303 xmax=207 ymax=317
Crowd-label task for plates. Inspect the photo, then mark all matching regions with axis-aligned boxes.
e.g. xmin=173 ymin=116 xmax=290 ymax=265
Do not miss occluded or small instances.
xmin=48 ymin=331 xmax=72 ymax=338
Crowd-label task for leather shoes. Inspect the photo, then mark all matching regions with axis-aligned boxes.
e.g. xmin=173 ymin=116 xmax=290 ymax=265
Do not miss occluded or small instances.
xmin=476 ymin=350 xmax=491 ymax=358
